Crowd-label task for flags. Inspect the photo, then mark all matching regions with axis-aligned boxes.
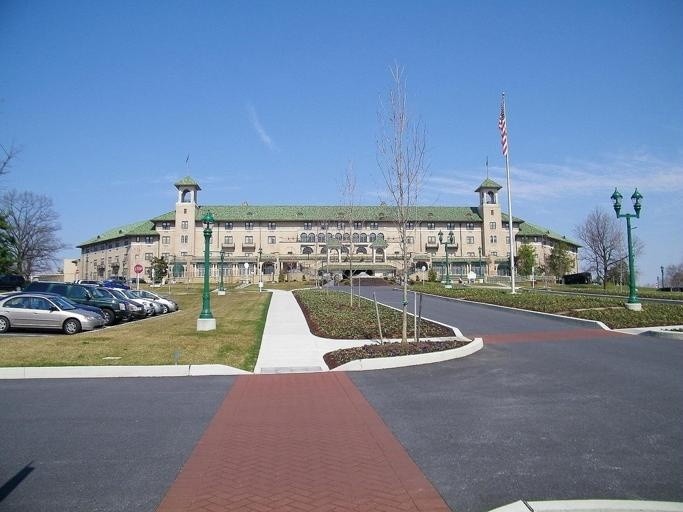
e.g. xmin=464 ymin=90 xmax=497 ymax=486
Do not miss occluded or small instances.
xmin=498 ymin=97 xmax=508 ymax=156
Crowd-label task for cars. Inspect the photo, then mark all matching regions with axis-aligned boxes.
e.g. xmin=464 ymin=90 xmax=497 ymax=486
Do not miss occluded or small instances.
xmin=0 ymin=276 xmax=178 ymax=335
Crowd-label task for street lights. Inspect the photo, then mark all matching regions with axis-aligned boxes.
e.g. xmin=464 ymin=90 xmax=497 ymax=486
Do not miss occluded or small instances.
xmin=610 ymin=187 xmax=643 ymax=303
xmin=478 ymin=245 xmax=482 ymax=278
xmin=219 ymin=250 xmax=225 ymax=291
xmin=199 ymin=209 xmax=215 ymax=319
xmin=438 ymin=229 xmax=455 ymax=285
xmin=660 ymin=265 xmax=664 ymax=288
